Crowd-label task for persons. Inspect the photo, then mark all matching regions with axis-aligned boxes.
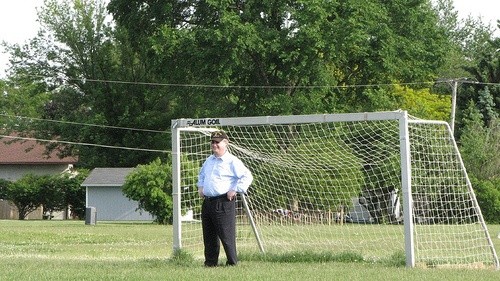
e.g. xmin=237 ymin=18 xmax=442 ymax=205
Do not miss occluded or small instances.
xmin=197 ymin=130 xmax=254 ymax=268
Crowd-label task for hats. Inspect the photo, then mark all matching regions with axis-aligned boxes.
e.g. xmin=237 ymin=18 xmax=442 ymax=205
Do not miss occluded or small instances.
xmin=209 ymin=131 xmax=227 ymax=142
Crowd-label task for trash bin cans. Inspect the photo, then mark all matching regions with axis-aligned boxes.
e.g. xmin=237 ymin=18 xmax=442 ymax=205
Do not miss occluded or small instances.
xmin=85 ymin=207 xmax=96 ymax=225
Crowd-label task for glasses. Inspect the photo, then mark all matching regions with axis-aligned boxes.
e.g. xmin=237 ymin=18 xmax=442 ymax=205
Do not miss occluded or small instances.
xmin=211 ymin=141 xmax=219 ymax=144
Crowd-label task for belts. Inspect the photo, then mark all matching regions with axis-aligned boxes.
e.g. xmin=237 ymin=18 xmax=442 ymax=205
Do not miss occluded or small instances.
xmin=205 ymin=194 xmax=227 ymax=200
